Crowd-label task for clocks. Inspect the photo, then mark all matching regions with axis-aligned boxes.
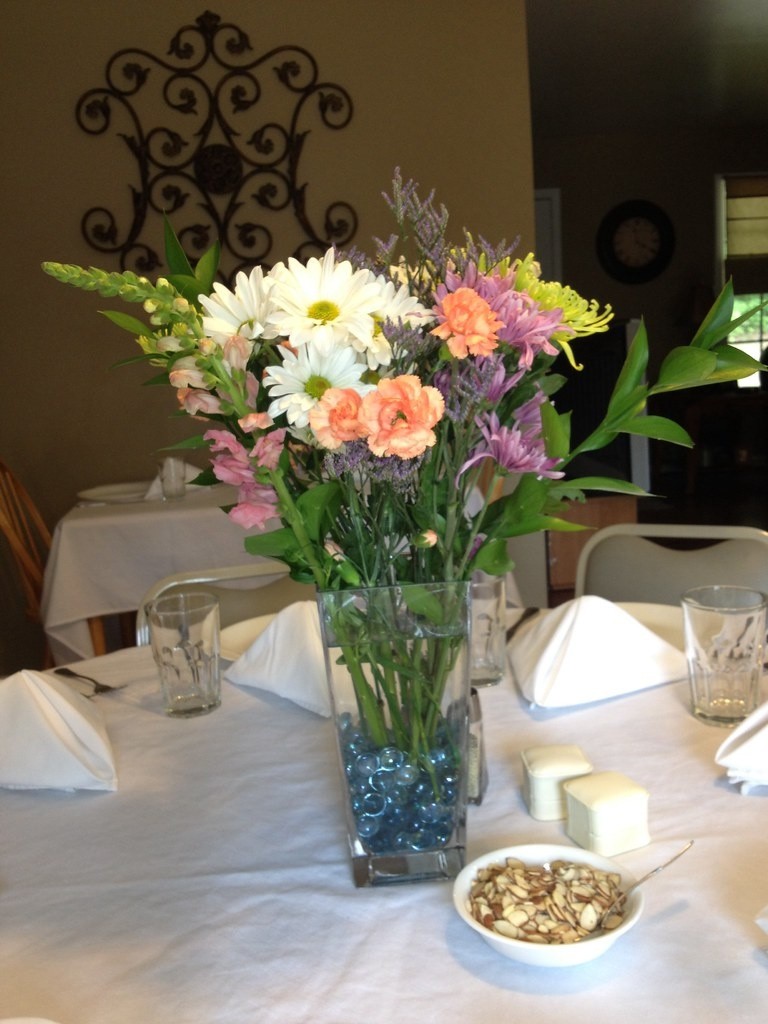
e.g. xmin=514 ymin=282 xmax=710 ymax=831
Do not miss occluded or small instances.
xmin=594 ymin=199 xmax=675 ymax=283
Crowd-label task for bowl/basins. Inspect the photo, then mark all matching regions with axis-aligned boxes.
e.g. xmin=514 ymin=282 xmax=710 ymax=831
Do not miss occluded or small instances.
xmin=453 ymin=844 xmax=645 ymax=967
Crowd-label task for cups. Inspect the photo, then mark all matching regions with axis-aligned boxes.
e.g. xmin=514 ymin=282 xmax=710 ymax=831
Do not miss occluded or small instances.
xmin=157 ymin=456 xmax=187 ymax=503
xmin=468 ymin=571 xmax=507 ymax=687
xmin=143 ymin=592 xmax=222 ymax=718
xmin=681 ymin=585 xmax=768 ymax=728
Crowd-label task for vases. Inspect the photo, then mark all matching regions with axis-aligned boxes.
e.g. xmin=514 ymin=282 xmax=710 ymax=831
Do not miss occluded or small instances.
xmin=317 ymin=580 xmax=470 ymax=888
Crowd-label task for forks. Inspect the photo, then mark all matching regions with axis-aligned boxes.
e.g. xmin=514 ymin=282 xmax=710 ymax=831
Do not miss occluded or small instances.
xmin=54 ymin=668 xmax=128 ymax=695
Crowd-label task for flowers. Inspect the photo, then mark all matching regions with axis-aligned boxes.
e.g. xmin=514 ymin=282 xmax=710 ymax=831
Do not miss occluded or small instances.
xmin=35 ymin=162 xmax=763 ymax=816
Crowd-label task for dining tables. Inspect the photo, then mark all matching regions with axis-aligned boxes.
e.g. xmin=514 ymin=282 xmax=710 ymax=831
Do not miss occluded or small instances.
xmin=0 ymin=600 xmax=768 ymax=1019
xmin=39 ymin=486 xmax=280 ymax=662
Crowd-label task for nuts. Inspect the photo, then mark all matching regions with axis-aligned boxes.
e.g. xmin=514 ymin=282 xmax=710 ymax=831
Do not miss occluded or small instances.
xmin=468 ymin=856 xmax=626 ymax=944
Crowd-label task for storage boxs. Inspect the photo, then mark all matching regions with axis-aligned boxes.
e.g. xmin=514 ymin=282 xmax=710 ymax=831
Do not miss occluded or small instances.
xmin=544 ymin=455 xmax=638 ymax=593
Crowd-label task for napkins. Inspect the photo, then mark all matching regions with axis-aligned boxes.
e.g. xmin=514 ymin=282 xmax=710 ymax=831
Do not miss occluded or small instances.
xmin=1 ymin=665 xmax=117 ymax=793
xmin=714 ymin=701 xmax=768 ymax=789
xmin=223 ymin=598 xmax=342 ymax=716
xmin=501 ymin=590 xmax=691 ymax=712
xmin=145 ymin=458 xmax=201 ymax=499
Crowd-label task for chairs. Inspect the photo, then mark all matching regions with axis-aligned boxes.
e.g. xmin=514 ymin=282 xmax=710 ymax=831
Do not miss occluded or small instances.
xmin=137 ymin=560 xmax=326 ymax=650
xmin=577 ymin=526 xmax=767 ymax=608
xmin=0 ymin=460 xmax=134 ymax=667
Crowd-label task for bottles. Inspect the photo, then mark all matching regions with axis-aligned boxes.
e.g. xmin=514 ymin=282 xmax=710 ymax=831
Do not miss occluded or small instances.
xmin=468 ymin=690 xmax=488 ymax=805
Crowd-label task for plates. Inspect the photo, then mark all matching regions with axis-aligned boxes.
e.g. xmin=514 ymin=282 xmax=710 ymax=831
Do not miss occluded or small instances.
xmin=75 ymin=482 xmax=153 ymax=502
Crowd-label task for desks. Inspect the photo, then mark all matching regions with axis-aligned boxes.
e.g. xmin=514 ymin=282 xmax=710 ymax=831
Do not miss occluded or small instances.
xmin=679 ymin=387 xmax=762 ymax=505
